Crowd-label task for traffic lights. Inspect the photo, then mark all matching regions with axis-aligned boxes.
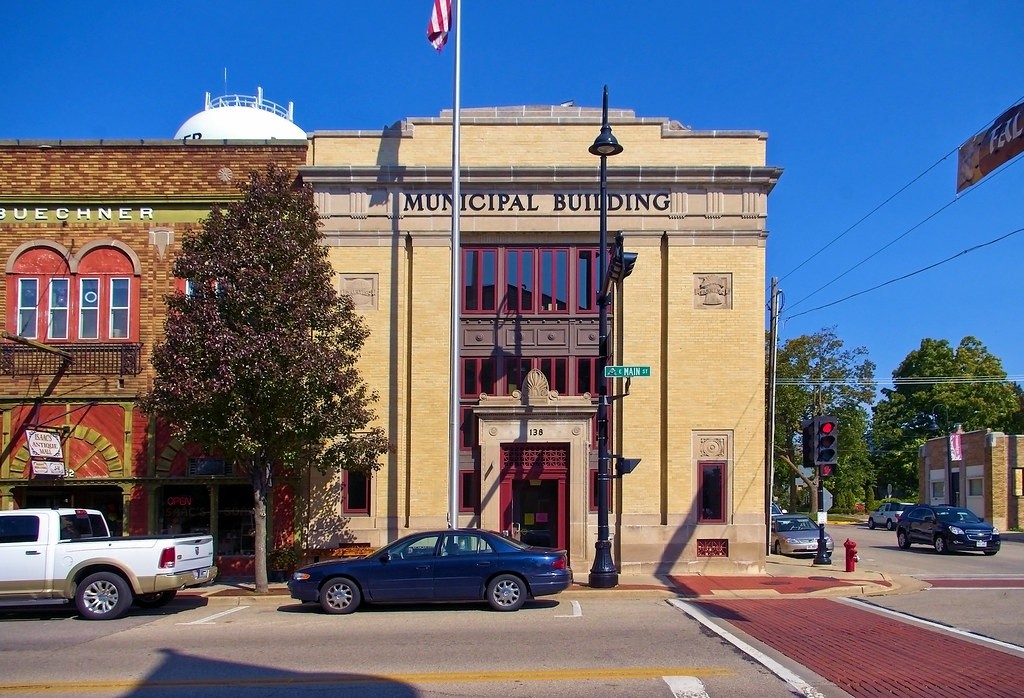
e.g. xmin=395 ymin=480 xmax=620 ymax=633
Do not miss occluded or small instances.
xmin=819 ymin=464 xmax=837 ymax=477
xmin=607 ymin=252 xmax=639 ymax=288
xmin=615 ymin=459 xmax=641 ymax=475
xmin=814 ymin=416 xmax=837 ymax=466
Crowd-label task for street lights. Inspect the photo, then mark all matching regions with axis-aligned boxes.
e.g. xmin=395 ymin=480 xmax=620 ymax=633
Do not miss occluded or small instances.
xmin=586 ymin=83 xmax=628 ymax=591
xmin=928 ymin=403 xmax=954 ymax=507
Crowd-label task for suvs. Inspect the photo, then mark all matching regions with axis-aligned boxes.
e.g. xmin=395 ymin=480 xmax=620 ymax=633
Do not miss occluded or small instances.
xmin=867 ymin=502 xmax=917 ymax=531
xmin=896 ymin=505 xmax=1002 ymax=557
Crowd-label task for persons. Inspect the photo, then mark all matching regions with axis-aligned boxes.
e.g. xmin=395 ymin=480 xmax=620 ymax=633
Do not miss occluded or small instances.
xmin=949 ymin=432 xmax=963 ymax=462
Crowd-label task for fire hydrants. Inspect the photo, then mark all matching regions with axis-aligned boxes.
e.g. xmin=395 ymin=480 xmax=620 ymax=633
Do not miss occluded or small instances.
xmin=843 ymin=538 xmax=860 ymax=572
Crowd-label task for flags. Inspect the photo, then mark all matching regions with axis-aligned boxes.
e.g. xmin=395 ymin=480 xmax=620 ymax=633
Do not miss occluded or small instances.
xmin=426 ymin=0 xmax=452 ymax=52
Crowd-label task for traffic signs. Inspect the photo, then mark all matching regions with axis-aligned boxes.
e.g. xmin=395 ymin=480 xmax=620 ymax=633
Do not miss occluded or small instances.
xmin=604 ymin=365 xmax=652 ymax=379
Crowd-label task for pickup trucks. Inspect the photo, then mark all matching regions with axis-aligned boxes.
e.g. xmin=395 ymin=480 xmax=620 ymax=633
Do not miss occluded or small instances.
xmin=0 ymin=507 xmax=219 ymax=623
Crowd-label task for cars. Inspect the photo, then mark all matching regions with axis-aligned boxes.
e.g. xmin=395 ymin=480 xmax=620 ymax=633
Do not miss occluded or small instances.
xmin=287 ymin=527 xmax=572 ymax=616
xmin=771 ymin=502 xmax=791 ymax=531
xmin=771 ymin=514 xmax=834 ymax=558
